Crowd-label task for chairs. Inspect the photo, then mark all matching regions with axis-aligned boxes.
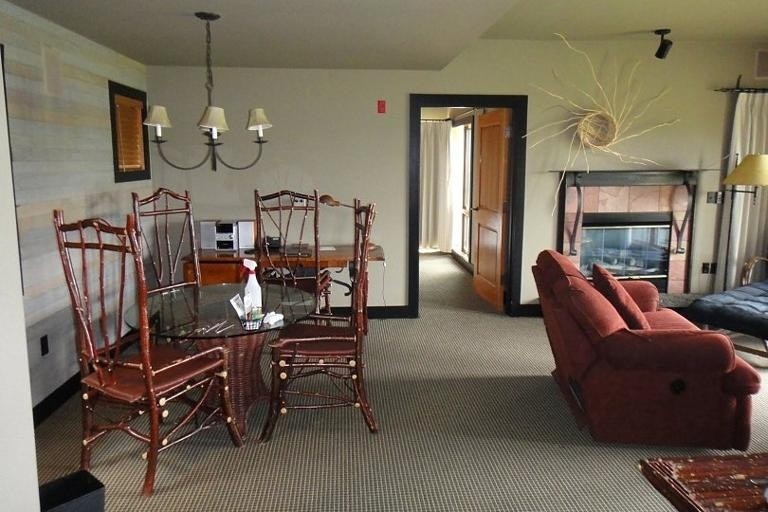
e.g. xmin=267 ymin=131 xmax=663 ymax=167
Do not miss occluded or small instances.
xmin=688 ymin=256 xmax=768 ymax=361
xmin=532 ymin=250 xmax=761 ymax=451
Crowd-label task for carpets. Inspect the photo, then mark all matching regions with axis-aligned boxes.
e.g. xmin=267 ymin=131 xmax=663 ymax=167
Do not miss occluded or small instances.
xmin=634 ymin=452 xmax=768 ymax=512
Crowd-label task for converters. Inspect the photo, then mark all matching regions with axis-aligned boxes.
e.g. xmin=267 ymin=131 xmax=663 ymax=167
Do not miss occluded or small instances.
xmin=383 ymin=262 xmax=386 ymax=267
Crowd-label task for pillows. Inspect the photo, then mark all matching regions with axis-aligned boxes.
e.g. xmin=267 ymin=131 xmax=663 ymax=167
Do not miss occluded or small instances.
xmin=593 ymin=263 xmax=652 ymax=330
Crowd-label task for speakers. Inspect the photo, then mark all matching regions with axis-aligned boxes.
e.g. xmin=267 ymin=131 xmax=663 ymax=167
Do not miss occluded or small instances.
xmin=200 ymin=220 xmax=218 ymax=250
xmin=236 ymin=220 xmax=257 ymax=249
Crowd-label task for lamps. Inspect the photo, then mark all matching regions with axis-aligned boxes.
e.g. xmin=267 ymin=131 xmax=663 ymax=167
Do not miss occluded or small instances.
xmin=141 ymin=11 xmax=274 ymax=172
xmin=654 ymin=28 xmax=673 ymax=60
xmin=720 ymin=154 xmax=768 ymax=198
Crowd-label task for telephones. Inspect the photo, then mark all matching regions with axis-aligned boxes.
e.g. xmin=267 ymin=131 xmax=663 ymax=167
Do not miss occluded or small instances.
xmin=262 ymin=236 xmax=280 ymax=249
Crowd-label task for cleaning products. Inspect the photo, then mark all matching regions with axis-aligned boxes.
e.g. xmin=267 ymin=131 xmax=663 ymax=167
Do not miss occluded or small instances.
xmin=240 ymin=258 xmax=263 ymax=316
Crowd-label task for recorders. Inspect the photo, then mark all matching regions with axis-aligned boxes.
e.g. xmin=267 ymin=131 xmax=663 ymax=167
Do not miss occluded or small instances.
xmin=216 ymin=220 xmax=238 ymax=251
